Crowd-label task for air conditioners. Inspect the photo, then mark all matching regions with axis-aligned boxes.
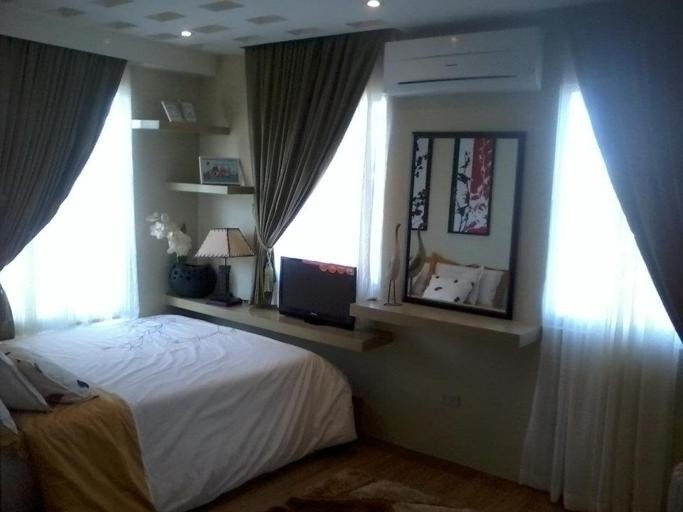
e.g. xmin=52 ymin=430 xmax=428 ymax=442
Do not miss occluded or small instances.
xmin=379 ymin=23 xmax=545 ymax=99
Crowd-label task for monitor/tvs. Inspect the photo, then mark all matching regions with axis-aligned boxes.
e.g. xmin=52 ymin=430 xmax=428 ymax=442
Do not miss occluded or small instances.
xmin=278 ymin=256 xmax=357 ymax=332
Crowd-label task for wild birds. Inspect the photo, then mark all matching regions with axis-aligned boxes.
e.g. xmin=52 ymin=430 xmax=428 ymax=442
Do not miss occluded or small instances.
xmin=385 ymin=223 xmax=402 ymax=305
xmin=406 ymin=221 xmax=428 ymax=297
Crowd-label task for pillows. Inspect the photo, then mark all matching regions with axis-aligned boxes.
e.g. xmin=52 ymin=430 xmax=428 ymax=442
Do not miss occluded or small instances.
xmin=408 ymin=255 xmax=509 ymax=306
xmin=0 ymin=284 xmax=99 ymax=435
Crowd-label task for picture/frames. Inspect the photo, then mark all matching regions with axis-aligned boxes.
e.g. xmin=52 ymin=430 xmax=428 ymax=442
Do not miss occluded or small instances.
xmin=448 ymin=136 xmax=496 ymax=236
xmin=411 ymin=137 xmax=434 ymax=231
xmin=198 ymin=155 xmax=242 ymax=187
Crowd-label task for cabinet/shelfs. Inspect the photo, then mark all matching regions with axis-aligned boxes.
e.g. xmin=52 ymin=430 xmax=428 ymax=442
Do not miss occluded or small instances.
xmin=131 ymin=118 xmax=255 ymax=196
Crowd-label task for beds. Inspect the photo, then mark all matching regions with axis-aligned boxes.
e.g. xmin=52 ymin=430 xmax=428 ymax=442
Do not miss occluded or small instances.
xmin=0 ymin=313 xmax=358 ymax=512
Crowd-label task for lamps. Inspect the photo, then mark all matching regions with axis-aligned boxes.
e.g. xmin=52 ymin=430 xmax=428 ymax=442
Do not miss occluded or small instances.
xmin=192 ymin=227 xmax=256 ymax=308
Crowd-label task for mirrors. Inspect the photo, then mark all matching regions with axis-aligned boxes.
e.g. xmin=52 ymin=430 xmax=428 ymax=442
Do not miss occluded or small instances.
xmin=400 ymin=130 xmax=527 ymax=322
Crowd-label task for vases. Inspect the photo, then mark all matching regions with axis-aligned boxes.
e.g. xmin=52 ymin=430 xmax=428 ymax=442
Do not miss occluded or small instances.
xmin=167 ymin=262 xmax=218 ymax=299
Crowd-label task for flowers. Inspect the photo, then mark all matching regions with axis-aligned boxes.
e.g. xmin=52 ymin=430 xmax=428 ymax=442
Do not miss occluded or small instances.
xmin=144 ymin=209 xmax=194 ymax=265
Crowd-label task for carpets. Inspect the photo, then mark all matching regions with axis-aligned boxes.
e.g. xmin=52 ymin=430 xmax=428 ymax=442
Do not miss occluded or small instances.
xmin=299 ymin=465 xmax=442 ymax=512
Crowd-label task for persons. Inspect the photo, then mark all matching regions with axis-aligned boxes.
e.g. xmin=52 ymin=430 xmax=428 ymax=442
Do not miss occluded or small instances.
xmin=206 ymin=161 xmax=227 ymax=176
